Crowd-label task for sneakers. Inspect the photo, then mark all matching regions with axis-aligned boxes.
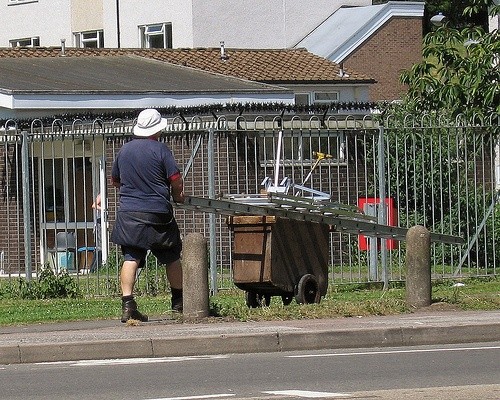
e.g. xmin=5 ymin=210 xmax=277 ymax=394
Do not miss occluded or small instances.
xmin=121 ymin=299 xmax=148 ymax=323
xmin=171 ymin=296 xmax=183 ymax=310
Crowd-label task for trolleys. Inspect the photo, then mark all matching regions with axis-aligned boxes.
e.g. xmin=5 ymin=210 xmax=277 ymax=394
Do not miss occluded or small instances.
xmin=226 ymin=217 xmax=329 ymax=309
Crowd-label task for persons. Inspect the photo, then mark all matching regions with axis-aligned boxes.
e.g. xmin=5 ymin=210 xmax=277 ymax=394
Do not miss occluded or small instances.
xmin=111 ymin=109 xmax=185 ymax=322
xmin=89 ymin=192 xmax=102 ymax=272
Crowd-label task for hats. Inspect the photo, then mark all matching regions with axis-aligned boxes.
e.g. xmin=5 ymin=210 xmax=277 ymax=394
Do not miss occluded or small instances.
xmin=133 ymin=109 xmax=167 ymax=137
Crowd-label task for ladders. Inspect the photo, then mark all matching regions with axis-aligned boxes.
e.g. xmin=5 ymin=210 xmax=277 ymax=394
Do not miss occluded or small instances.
xmin=171 ymin=193 xmax=465 ymax=246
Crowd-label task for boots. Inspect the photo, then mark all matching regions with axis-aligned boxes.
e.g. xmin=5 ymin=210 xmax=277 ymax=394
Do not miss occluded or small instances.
xmin=90 ymin=250 xmax=102 ymax=274
